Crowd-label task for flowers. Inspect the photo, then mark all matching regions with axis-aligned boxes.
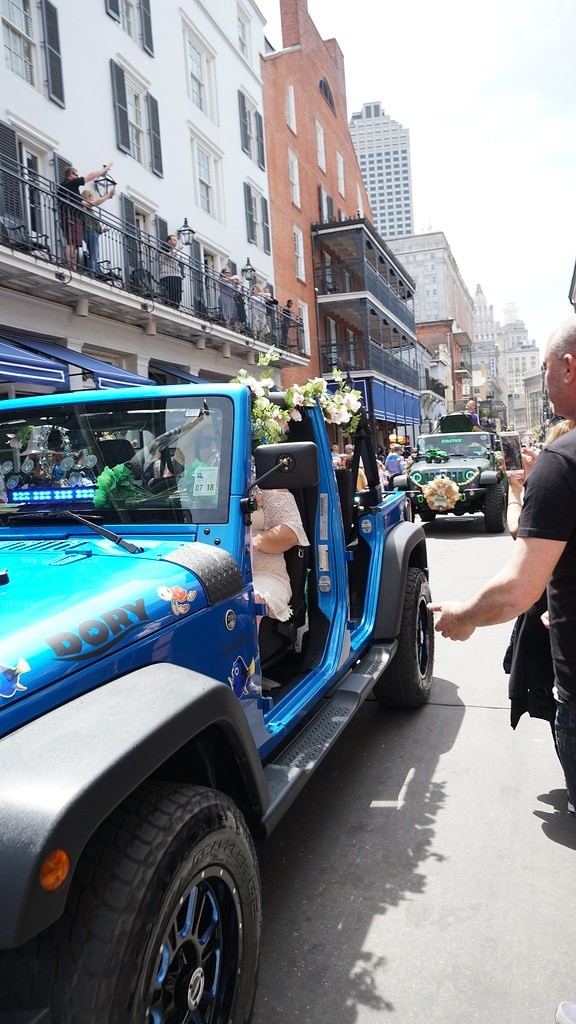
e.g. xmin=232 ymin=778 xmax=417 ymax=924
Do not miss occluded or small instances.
xmin=420 ymin=475 xmax=461 ymax=511
xmin=494 ymin=450 xmax=507 ymax=475
xmin=92 ymin=458 xmax=221 ymax=509
xmin=226 ymin=344 xmax=367 ymax=445
xmin=5 ymin=426 xmax=33 ymax=450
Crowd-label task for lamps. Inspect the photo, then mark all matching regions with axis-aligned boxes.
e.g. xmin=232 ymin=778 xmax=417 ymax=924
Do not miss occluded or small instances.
xmin=247 ymin=351 xmax=255 ymax=365
xmin=196 ymin=337 xmax=205 ymax=350
xmin=222 ymin=344 xmax=231 ymax=358
xmin=94 ymin=164 xmax=117 ymax=199
xmin=75 ymin=298 xmax=89 ymax=317
xmin=177 ymin=218 xmax=195 ymax=245
xmin=144 ymin=322 xmax=156 ymax=336
xmin=242 ymin=256 xmax=255 ymax=280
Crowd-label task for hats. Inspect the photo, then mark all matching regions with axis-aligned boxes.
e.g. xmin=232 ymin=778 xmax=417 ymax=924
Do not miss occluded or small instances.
xmin=174 ymin=410 xmax=215 ymax=467
xmin=18 ymin=425 xmax=72 ymax=457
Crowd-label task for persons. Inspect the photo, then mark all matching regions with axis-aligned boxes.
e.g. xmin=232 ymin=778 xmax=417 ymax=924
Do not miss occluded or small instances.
xmin=156 ymin=235 xmax=184 ymax=310
xmin=331 ymin=443 xmax=412 ymax=491
xmin=218 ymin=266 xmax=293 ymax=350
xmin=58 ymin=162 xmax=117 ymax=271
xmin=424 ymin=313 xmax=576 ymax=817
xmin=174 ymin=408 xmax=311 ymax=640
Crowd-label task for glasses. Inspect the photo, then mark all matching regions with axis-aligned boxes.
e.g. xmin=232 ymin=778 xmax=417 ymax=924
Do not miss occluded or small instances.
xmin=198 ymin=434 xmax=222 ymax=448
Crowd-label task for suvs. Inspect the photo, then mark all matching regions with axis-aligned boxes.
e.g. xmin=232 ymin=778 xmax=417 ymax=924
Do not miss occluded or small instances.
xmin=401 ymin=412 xmax=510 ymax=535
xmin=0 ymin=385 xmax=438 ymax=1024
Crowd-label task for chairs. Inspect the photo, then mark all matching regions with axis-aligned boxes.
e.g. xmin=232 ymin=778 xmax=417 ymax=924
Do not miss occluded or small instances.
xmin=131 ymin=269 xmax=171 ymax=306
xmin=77 ymin=249 xmax=125 ymax=290
xmin=0 ymin=216 xmax=53 ymax=261
xmin=251 ymin=440 xmax=319 ymax=644
xmin=195 ymin=297 xmax=225 ymax=326
